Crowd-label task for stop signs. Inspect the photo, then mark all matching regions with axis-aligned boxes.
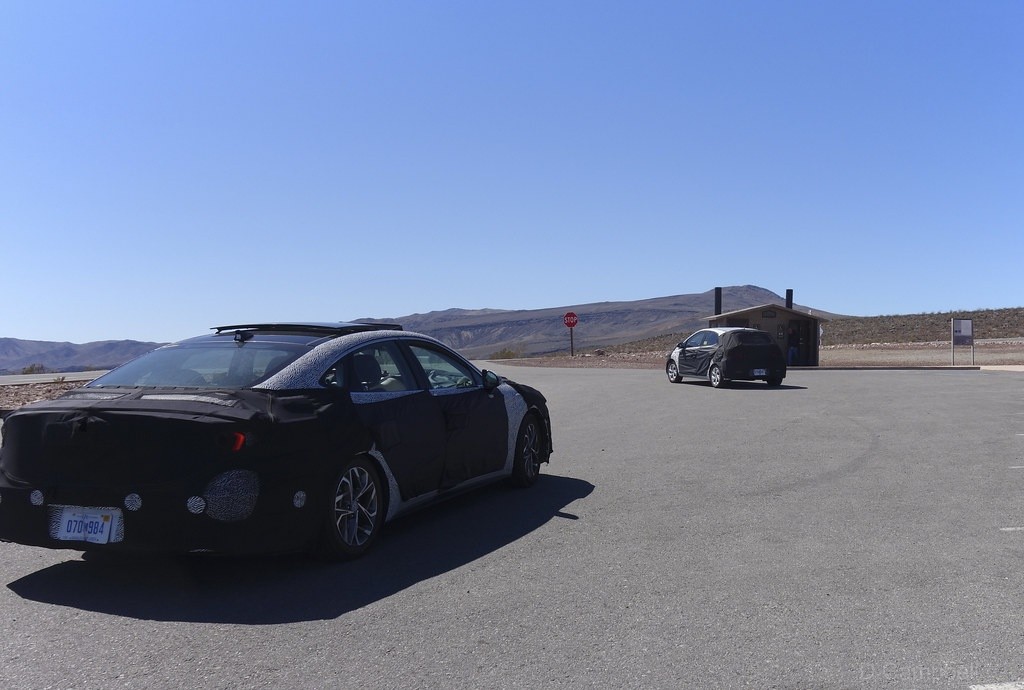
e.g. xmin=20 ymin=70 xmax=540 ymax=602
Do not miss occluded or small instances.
xmin=563 ymin=312 xmax=578 ymax=328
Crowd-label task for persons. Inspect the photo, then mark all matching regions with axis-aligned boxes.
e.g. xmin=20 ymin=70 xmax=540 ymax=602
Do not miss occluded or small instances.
xmin=788 ymin=329 xmax=800 ymax=366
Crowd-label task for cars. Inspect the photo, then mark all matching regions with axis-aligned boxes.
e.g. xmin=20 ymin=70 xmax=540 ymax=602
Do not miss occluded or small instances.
xmin=666 ymin=326 xmax=787 ymax=388
xmin=0 ymin=322 xmax=554 ymax=573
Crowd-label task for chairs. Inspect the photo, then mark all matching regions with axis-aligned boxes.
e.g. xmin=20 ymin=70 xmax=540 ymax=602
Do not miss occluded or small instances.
xmin=143 ymin=368 xmax=206 ymax=386
xmin=264 ymin=356 xmax=291 ymax=376
xmin=355 ymin=353 xmax=408 ymax=393
